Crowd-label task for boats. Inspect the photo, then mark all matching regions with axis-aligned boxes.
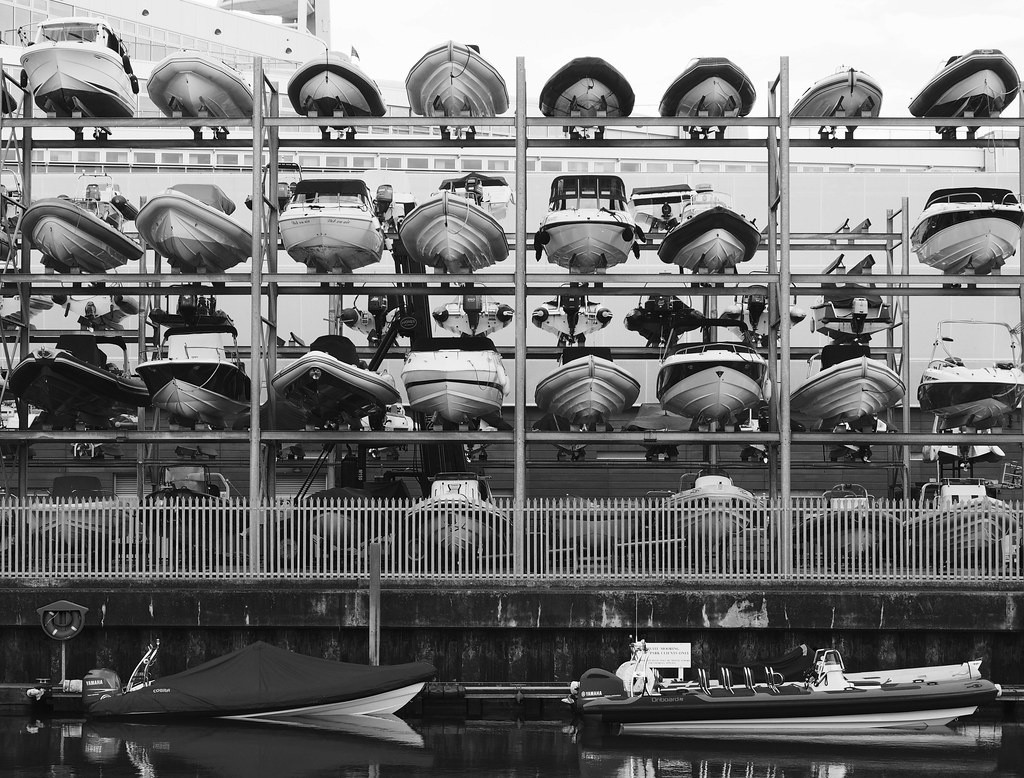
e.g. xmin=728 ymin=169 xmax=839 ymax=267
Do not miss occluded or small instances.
xmin=651 ymin=464 xmax=766 ymax=564
xmin=909 ymin=187 xmax=1024 ymax=294
xmin=280 ymin=443 xmax=514 ymax=575
xmin=560 ymin=642 xmax=1001 ymax=733
xmin=17 ymin=17 xmax=140 ymax=142
xmin=430 ymin=286 xmax=514 ymax=341
xmin=579 ymin=711 xmax=1002 ymax=777
xmin=657 ymin=56 xmax=755 ymax=141
xmin=4 ymin=334 xmax=151 ymax=432
xmin=533 ymin=174 xmax=646 ymax=289
xmin=530 ymin=283 xmax=615 ymax=348
xmin=338 ymin=295 xmax=413 ymax=348
xmin=805 ymin=254 xmax=894 ymax=339
xmin=90 ymin=712 xmax=434 ymax=778
xmin=135 ymin=462 xmax=250 ymax=546
xmin=146 ymin=51 xmax=255 ymax=140
xmin=916 ymin=320 xmax=1024 ymax=434
xmin=269 ymin=334 xmax=402 ymax=431
xmin=54 ymin=292 xmax=140 ymax=333
xmin=906 ymin=47 xmax=1021 ymax=139
xmin=285 ymin=51 xmax=386 ymax=140
xmin=133 ymin=182 xmax=253 ymax=286
xmin=28 ymin=476 xmax=131 ymax=558
xmin=398 ymin=174 xmax=509 ymax=285
xmin=403 ymin=336 xmax=507 ymax=430
xmin=657 ymin=344 xmax=775 ymax=436
xmin=788 ymin=68 xmax=883 ymax=140
xmin=799 ymin=444 xmax=1023 ymax=576
xmin=403 ymin=40 xmax=511 ymax=141
xmin=135 ymin=293 xmax=251 ymax=432
xmin=17 ymin=175 xmax=143 ymax=287
xmin=658 ymin=184 xmax=762 ymax=286
xmin=26 ymin=638 xmax=161 ymax=716
xmin=718 ymin=270 xmax=807 ymax=347
xmin=534 ymin=347 xmax=642 ymax=431
xmin=82 ymin=640 xmax=437 ymax=724
xmin=537 ymin=55 xmax=636 ymax=143
xmin=789 ymin=344 xmax=906 ymax=433
xmin=277 ymin=178 xmax=395 ymax=285
